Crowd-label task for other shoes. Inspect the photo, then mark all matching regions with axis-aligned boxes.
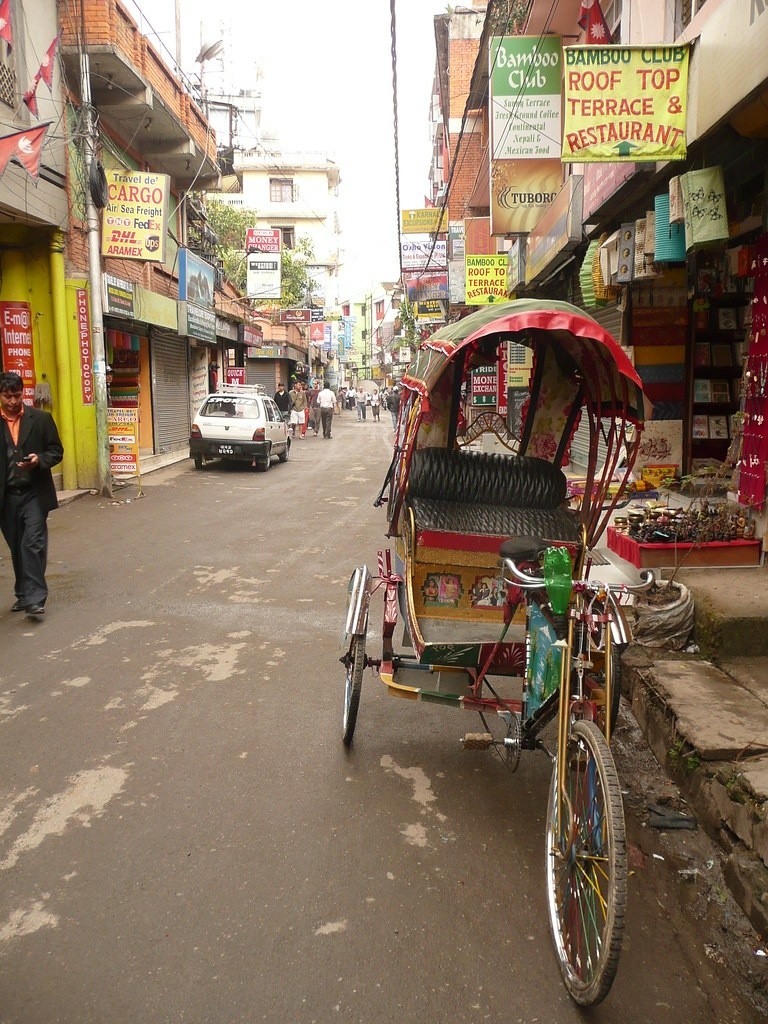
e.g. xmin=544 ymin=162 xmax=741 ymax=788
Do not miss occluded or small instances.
xmin=299 ymin=434 xmax=305 ymax=439
xmin=324 ymin=435 xmax=326 ymax=438
xmin=314 ymin=432 xmax=317 ymax=436
xmin=378 ymin=417 xmax=380 ymax=421
xmin=358 ymin=419 xmax=360 ymax=422
xmin=291 ymin=431 xmax=295 ymax=438
xmin=363 ymin=418 xmax=366 ymax=422
xmin=12 ymin=600 xmax=24 ymax=611
xmin=326 ymin=432 xmax=332 ymax=439
xmin=373 ymin=417 xmax=376 ymax=421
xmin=26 ymin=605 xmax=45 ymax=614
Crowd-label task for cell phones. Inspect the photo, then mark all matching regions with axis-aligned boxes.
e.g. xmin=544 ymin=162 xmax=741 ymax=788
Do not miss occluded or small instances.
xmin=23 ymin=457 xmax=32 ymax=463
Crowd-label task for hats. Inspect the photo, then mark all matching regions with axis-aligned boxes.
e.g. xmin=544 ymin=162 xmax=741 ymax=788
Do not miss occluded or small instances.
xmin=393 ymin=386 xmax=398 ymax=391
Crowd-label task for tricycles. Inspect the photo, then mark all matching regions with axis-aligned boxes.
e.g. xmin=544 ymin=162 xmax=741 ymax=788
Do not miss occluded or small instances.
xmin=341 ymin=297 xmax=657 ymax=1010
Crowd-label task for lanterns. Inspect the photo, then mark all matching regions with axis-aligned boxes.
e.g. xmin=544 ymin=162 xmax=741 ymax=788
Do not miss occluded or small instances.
xmin=579 ymin=163 xmax=730 ymax=309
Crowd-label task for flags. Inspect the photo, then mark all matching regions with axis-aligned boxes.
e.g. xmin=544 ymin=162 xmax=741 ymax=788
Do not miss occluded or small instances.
xmin=577 ymin=0 xmax=613 ymax=44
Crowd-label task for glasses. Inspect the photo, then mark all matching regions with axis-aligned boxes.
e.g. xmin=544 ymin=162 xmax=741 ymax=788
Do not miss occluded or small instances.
xmin=313 ymin=383 xmax=316 ymax=385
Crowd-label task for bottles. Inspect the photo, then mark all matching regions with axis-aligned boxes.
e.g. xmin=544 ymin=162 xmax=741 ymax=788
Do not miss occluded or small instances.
xmin=543 ymin=545 xmax=572 ymax=615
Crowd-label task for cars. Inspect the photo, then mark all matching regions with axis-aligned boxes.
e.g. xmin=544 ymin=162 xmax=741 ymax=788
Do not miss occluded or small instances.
xmin=188 ymin=383 xmax=291 ymax=471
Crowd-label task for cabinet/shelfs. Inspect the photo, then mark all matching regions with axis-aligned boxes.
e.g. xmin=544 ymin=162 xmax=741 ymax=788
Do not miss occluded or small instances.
xmin=682 ymin=245 xmax=761 ymax=480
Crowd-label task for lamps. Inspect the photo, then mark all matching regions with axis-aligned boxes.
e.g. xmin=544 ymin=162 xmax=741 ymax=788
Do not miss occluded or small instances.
xmin=169 ymin=39 xmax=229 ymax=91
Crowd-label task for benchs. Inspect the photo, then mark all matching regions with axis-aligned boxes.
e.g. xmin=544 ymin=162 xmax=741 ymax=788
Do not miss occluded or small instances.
xmin=404 ymin=446 xmax=585 ymax=576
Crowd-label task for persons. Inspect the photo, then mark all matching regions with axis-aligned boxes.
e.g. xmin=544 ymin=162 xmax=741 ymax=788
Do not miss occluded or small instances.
xmin=308 ymin=379 xmax=322 ymax=436
xmin=380 ymin=386 xmax=400 ymax=432
xmin=425 ymin=577 xmax=509 ymax=607
xmin=316 ymin=381 xmax=338 ymax=439
xmin=300 ymin=380 xmax=311 ymax=437
xmin=288 ymin=380 xmax=308 ymax=440
xmin=338 ymin=386 xmax=356 ymax=411
xmin=355 ymin=387 xmax=367 ymax=422
xmin=106 ymin=366 xmax=113 ymax=408
xmin=0 ymin=372 xmax=64 ymax=613
xmin=371 ymin=389 xmax=382 ymax=422
xmin=273 ymin=382 xmax=293 ymax=416
